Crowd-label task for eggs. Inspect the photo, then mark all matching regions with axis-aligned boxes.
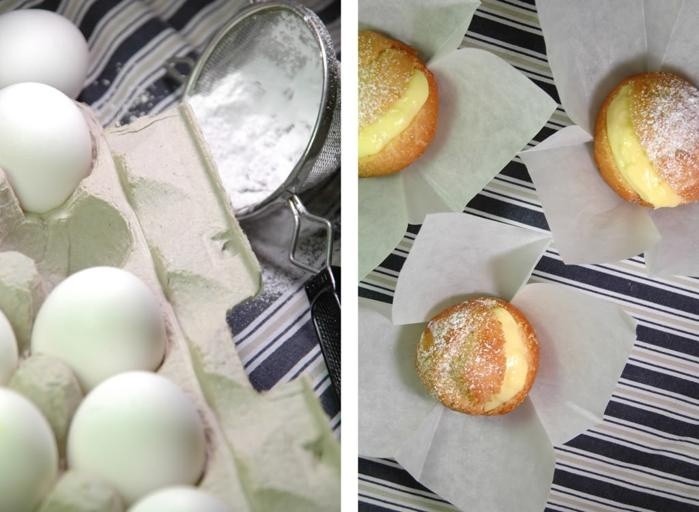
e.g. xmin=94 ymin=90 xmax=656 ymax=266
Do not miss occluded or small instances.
xmin=0 ymin=9 xmax=227 ymax=512
